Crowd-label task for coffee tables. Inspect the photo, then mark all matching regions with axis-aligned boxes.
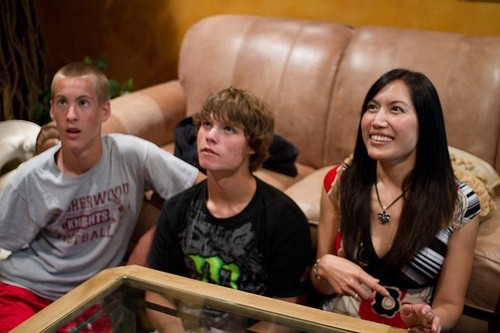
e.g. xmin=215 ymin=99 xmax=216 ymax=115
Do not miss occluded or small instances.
xmin=8 ymin=265 xmax=410 ymax=333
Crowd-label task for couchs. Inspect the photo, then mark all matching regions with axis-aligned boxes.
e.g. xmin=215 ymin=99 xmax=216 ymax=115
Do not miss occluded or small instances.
xmin=35 ymin=15 xmax=500 ymax=333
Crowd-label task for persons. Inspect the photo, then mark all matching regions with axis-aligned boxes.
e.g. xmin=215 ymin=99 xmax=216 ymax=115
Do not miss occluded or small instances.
xmin=311 ymin=69 xmax=480 ymax=333
xmin=145 ymin=85 xmax=314 ymax=333
xmin=0 ymin=62 xmax=209 ymax=333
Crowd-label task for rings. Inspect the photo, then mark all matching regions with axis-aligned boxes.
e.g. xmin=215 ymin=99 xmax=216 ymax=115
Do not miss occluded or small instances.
xmin=353 ymin=293 xmax=359 ymax=299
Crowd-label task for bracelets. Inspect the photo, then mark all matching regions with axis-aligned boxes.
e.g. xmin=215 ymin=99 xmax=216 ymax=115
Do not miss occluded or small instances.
xmin=314 ymin=258 xmax=328 ymax=284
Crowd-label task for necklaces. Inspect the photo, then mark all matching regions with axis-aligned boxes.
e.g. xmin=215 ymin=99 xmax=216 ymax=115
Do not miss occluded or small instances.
xmin=374 ymin=178 xmax=410 ymax=226
